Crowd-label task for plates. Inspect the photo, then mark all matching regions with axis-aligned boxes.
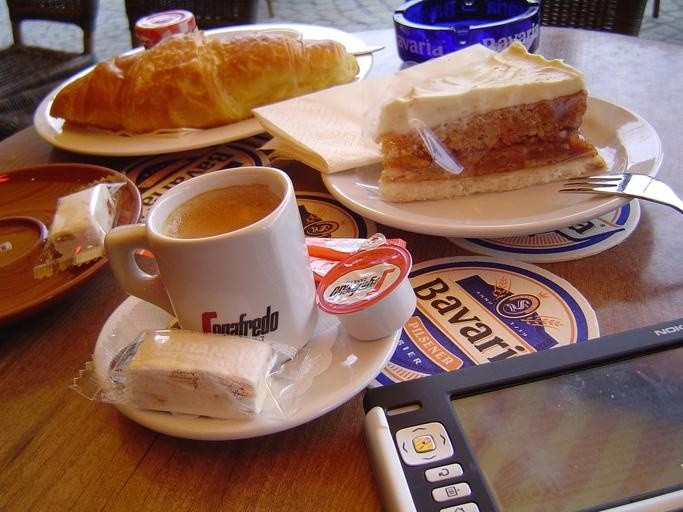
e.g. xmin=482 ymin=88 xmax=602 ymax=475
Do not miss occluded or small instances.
xmin=0 ymin=164 xmax=143 ymax=322
xmin=33 ymin=22 xmax=374 ymax=157
xmin=93 ymin=293 xmax=401 ymax=442
xmin=319 ymin=98 xmax=664 ymax=240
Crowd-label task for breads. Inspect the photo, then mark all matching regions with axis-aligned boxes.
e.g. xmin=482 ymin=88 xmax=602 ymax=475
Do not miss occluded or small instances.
xmin=50 ymin=34 xmax=361 ymax=130
xmin=48 ymin=184 xmax=118 ymax=255
xmin=128 ymin=331 xmax=274 ymax=419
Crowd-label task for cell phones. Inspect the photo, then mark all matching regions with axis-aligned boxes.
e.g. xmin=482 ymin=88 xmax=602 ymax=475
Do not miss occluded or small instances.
xmin=361 ymin=317 xmax=682 ymax=511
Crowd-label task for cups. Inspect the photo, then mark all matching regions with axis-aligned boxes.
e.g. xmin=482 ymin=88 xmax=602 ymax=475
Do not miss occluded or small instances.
xmin=105 ymin=165 xmax=319 ymax=370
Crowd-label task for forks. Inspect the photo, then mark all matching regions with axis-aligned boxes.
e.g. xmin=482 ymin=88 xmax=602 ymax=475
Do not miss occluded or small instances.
xmin=557 ymin=171 xmax=683 ymax=213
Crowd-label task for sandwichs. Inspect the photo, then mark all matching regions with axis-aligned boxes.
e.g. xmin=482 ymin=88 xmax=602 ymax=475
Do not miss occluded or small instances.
xmin=372 ymin=40 xmax=610 ymax=205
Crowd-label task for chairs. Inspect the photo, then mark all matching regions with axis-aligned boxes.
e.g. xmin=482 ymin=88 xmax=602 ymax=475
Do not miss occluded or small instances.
xmin=1 ymin=0 xmax=99 ymax=145
xmin=124 ymin=0 xmax=276 ymax=50
xmin=539 ymin=0 xmax=661 ymax=36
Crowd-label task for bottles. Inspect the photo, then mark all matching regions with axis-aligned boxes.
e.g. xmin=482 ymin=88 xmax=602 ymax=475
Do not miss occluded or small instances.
xmin=134 ymin=8 xmax=199 ymax=49
